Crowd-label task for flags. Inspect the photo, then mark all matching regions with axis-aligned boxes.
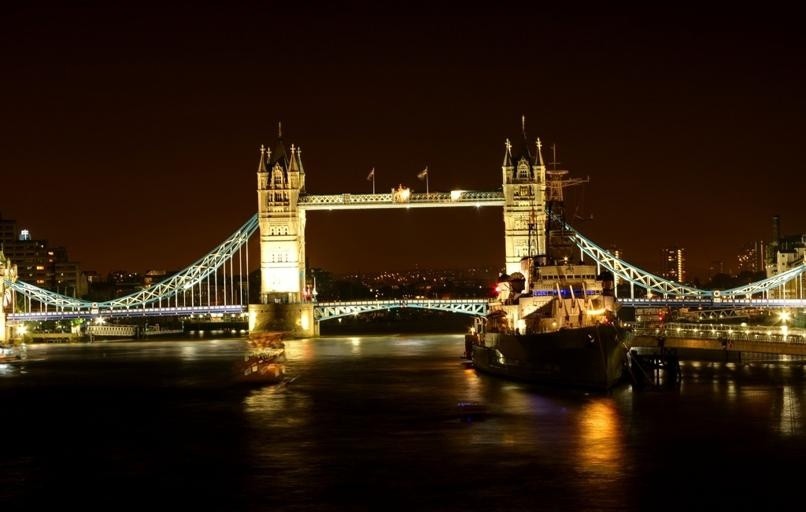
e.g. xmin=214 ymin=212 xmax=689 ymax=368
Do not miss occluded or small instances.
xmin=417 ymin=168 xmax=427 ymax=180
xmin=365 ymin=169 xmax=374 ymax=180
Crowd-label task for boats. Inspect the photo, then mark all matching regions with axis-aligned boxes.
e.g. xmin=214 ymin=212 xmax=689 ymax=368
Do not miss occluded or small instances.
xmin=237 ymin=328 xmax=291 ymax=382
xmin=463 ymin=185 xmax=636 ymax=398
